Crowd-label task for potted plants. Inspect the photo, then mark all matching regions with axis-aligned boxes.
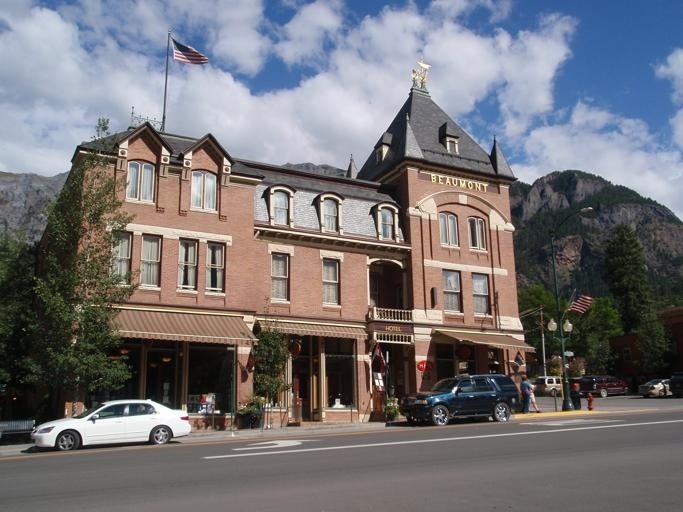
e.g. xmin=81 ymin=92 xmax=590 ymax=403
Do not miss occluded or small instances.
xmin=237 ymin=392 xmax=266 ymax=427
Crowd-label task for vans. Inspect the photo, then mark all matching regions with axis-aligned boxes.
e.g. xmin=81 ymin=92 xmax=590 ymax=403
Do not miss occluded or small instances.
xmin=537 ymin=375 xmax=562 ymax=397
xmin=580 ymin=374 xmax=632 ymax=398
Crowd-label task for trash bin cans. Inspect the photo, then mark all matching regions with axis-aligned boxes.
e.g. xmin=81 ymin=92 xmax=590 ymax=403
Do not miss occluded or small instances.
xmin=571 ymin=383 xmax=581 ymax=410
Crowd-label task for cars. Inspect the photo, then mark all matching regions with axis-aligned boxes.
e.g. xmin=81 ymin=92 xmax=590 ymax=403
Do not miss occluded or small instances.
xmin=30 ymin=398 xmax=193 ymax=452
xmin=638 ymin=379 xmax=673 ymax=398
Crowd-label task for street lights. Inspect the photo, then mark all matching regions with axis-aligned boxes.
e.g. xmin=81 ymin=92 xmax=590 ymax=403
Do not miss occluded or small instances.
xmin=548 ymin=205 xmax=595 ymax=412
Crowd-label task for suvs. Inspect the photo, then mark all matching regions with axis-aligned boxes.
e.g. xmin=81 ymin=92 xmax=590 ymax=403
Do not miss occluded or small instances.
xmin=399 ymin=373 xmax=521 ymax=426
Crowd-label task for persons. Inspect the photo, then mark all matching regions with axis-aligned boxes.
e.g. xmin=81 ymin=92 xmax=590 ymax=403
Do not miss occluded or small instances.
xmin=519 ymin=373 xmax=534 ymax=414
xmin=526 ymin=377 xmax=540 ymax=413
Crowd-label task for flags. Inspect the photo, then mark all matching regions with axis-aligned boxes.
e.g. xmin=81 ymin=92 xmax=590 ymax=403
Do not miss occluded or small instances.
xmin=168 ymin=35 xmax=209 ymax=65
xmin=566 ymin=290 xmax=594 ymax=317
xmin=510 ymin=350 xmax=524 ymax=374
xmin=370 ymin=342 xmax=385 ymax=374
